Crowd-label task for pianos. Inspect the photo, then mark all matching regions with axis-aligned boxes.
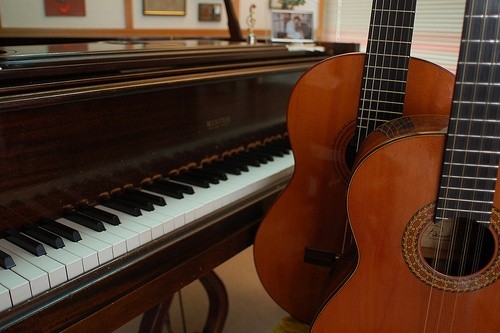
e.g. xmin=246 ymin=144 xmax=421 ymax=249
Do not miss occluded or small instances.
xmin=0 ymin=40 xmax=328 ymax=332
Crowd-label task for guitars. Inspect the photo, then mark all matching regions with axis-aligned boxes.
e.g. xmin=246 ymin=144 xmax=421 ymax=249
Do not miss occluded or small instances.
xmin=310 ymin=0 xmax=500 ymax=333
xmin=252 ymin=0 xmax=456 ymax=325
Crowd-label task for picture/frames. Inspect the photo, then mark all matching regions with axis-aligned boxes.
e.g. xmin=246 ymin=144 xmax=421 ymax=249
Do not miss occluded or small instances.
xmin=269 ymin=8 xmax=317 ymax=44
xmin=142 ymin=0 xmax=188 ymax=17
